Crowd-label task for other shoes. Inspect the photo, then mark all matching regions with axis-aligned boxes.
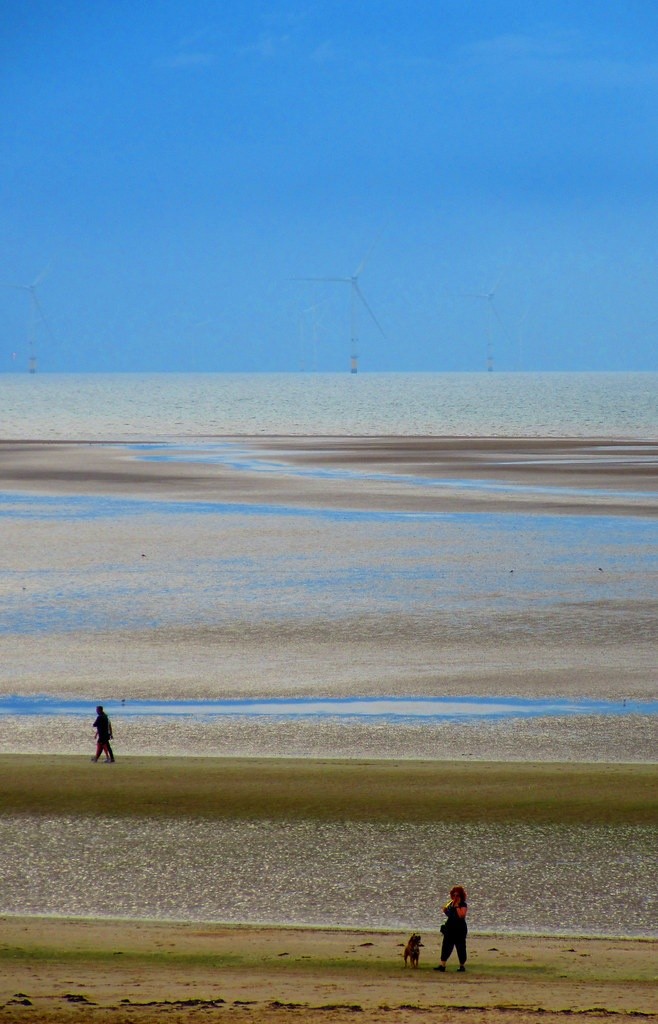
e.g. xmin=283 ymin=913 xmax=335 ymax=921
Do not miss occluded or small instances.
xmin=433 ymin=965 xmax=445 ymax=971
xmin=457 ymin=966 xmax=465 ymax=972
xmin=91 ymin=758 xmax=97 ymax=762
xmin=104 ymin=759 xmax=109 ymax=762
xmin=110 ymin=760 xmax=115 ymax=762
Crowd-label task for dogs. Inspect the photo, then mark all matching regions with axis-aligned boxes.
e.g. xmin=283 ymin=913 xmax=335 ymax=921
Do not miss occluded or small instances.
xmin=404 ymin=933 xmax=425 ymax=968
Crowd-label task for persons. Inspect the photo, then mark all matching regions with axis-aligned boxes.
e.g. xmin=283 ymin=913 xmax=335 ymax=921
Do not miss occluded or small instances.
xmin=90 ymin=705 xmax=117 ymax=764
xmin=90 ymin=718 xmax=114 ymax=762
xmin=432 ymin=886 xmax=469 ymax=973
